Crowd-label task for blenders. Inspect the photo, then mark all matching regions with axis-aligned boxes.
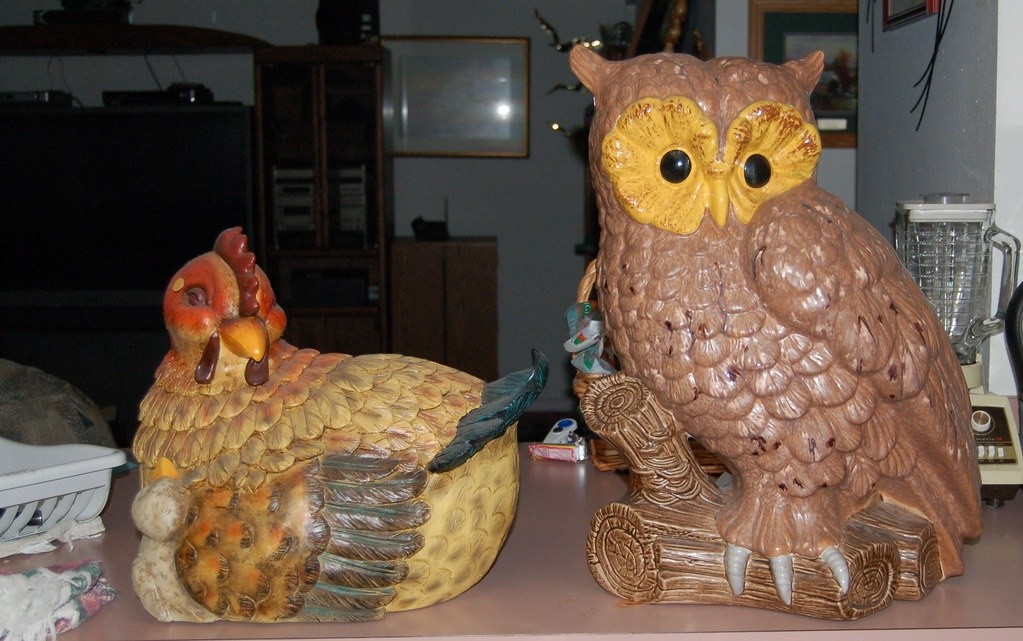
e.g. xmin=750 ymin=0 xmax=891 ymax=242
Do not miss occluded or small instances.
xmin=889 ymin=193 xmax=1023 ymax=503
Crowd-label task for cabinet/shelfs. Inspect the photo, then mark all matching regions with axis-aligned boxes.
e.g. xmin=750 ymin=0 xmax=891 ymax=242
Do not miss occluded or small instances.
xmin=0 ymin=441 xmax=1023 ymax=640
xmin=0 ymin=103 xmax=258 ymax=449
xmin=389 ymin=235 xmax=500 ymax=386
xmin=254 ymin=43 xmax=397 ymax=355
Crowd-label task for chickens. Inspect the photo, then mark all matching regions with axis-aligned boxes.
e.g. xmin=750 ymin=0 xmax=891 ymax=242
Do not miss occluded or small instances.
xmin=130 ymin=227 xmax=552 ymax=623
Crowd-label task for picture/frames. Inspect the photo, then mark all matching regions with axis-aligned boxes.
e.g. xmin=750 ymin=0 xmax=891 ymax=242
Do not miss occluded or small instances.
xmin=748 ymin=0 xmax=859 ymax=149
xmin=881 ymin=0 xmax=941 ymax=32
xmin=378 ymin=35 xmax=532 ymax=160
